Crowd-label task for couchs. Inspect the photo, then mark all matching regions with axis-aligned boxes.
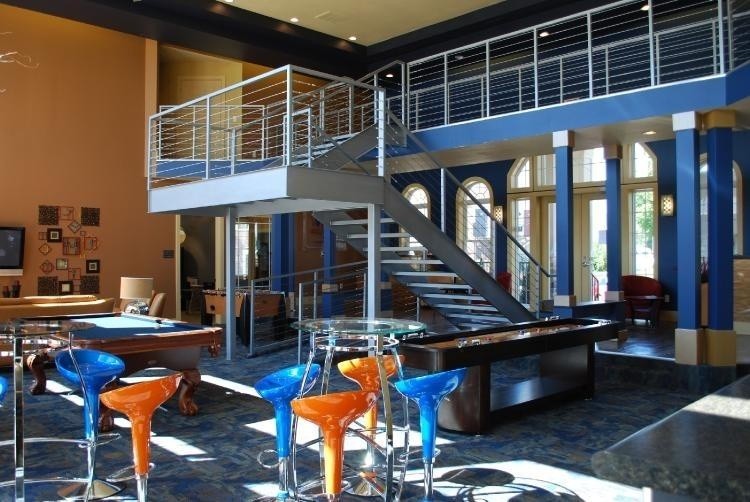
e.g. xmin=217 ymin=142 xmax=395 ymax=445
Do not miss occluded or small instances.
xmin=0 ymin=296 xmax=118 ymax=366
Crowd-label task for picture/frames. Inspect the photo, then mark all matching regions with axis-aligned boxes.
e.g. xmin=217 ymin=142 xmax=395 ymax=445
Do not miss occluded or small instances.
xmin=35 ymin=205 xmax=102 ymax=295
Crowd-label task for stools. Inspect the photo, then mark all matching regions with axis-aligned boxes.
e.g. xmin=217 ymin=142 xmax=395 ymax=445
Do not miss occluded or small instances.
xmin=397 ymin=367 xmax=466 ymax=502
xmin=54 ymin=349 xmax=124 ymax=502
xmin=291 ymin=392 xmax=381 ymax=502
xmin=254 ymin=361 xmax=321 ymax=502
xmin=101 ymin=373 xmax=183 ymax=502
xmin=0 ymin=374 xmax=10 ymax=408
xmin=339 ymin=354 xmax=405 ymax=495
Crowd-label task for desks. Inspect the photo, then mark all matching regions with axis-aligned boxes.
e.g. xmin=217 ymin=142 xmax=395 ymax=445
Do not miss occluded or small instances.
xmin=26 ymin=310 xmax=223 ymax=432
xmin=625 ymin=295 xmax=665 ymax=328
xmin=0 ymin=318 xmax=95 ymax=502
xmin=285 ymin=315 xmax=427 ymax=502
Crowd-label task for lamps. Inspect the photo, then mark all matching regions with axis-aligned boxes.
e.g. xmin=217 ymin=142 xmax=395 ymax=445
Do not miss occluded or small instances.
xmin=661 ymin=192 xmax=674 ymax=217
xmin=119 ymin=276 xmax=155 ymax=313
xmin=180 ymin=215 xmax=187 ymax=245
xmin=493 ymin=205 xmax=504 ymax=225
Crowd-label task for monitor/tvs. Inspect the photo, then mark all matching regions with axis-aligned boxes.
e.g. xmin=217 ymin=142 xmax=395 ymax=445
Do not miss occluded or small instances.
xmin=0 ymin=227 xmax=26 ymax=276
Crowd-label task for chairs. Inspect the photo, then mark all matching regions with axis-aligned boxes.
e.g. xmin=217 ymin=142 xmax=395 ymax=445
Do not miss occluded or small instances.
xmin=495 ymin=271 xmax=512 ymax=295
xmin=622 ymin=272 xmax=667 ymax=331
xmin=118 ymin=288 xmax=169 ymax=318
